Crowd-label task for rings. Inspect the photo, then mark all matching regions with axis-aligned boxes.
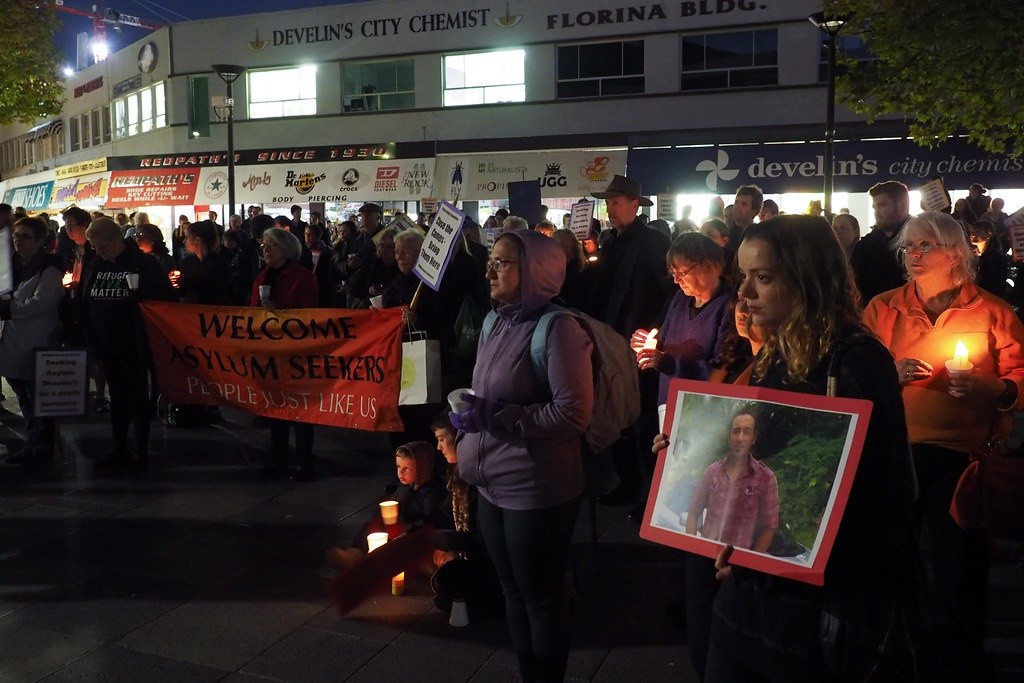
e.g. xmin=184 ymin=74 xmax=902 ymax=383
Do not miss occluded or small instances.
xmin=907 ymin=371 xmax=909 ymax=377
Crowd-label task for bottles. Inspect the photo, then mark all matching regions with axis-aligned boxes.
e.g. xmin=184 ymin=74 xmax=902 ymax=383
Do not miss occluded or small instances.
xmin=392 ymin=572 xmax=404 ymax=595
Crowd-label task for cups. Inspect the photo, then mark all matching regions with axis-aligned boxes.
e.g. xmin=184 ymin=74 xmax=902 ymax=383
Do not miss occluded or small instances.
xmin=638 ymin=338 xmax=657 ymax=362
xmin=945 ymin=361 xmax=974 ymax=374
xmin=369 ymin=295 xmax=383 ymax=309
xmin=125 ymin=274 xmax=139 ymax=289
xmin=258 ymin=285 xmax=271 ymax=300
xmin=62 ymin=273 xmax=72 ymax=286
xmin=366 ymin=533 xmax=389 ymax=554
xmin=378 ymin=500 xmax=399 ymax=525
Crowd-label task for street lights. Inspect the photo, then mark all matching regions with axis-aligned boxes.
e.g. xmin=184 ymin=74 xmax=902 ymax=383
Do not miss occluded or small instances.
xmin=212 ymin=62 xmax=249 ymax=229
xmin=808 ymin=10 xmax=861 ymax=221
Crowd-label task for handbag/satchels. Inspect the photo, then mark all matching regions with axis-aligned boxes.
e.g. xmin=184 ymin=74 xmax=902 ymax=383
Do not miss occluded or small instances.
xmin=159 ymin=405 xmax=209 ymax=428
xmin=950 ymin=412 xmax=1024 ymax=558
xmin=445 ymin=299 xmax=489 ymax=360
xmin=397 ymin=314 xmax=442 ymax=406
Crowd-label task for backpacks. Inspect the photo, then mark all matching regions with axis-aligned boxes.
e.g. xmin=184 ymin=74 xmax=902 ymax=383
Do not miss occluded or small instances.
xmin=482 ymin=304 xmax=642 ymax=454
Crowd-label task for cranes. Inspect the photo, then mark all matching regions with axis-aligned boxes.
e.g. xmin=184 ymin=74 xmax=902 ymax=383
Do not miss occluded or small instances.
xmin=35 ymin=0 xmax=170 ymax=59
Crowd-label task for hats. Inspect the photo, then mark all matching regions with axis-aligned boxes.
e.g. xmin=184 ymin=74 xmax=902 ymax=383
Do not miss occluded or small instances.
xmin=590 ymin=174 xmax=654 ymax=208
xmin=359 ymin=204 xmax=383 ymax=214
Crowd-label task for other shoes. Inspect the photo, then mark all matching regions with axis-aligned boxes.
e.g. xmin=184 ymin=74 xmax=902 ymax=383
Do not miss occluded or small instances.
xmin=288 ymin=456 xmax=314 ymax=481
xmin=2 ymin=448 xmax=51 ymax=464
xmin=624 ymin=508 xmax=642 ymax=523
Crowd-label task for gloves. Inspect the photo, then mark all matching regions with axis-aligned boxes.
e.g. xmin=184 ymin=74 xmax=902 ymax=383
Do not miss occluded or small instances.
xmin=447 ymin=393 xmax=495 ymax=433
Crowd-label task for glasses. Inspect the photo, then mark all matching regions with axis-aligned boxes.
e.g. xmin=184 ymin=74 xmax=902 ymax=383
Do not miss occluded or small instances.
xmin=12 ymin=232 xmax=38 ymax=242
xmin=485 ymin=259 xmax=519 ymax=273
xmin=133 ymin=231 xmax=151 ymax=237
xmin=898 ymin=240 xmax=956 ymax=254
xmin=667 ymin=264 xmax=698 ymax=277
xmin=260 ymin=241 xmax=276 ymax=248
xmin=90 ymin=238 xmax=114 ymax=251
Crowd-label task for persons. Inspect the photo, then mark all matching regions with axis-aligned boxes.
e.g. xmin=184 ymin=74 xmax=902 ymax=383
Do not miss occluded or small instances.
xmin=448 ymin=229 xmax=595 ymax=683
xmin=0 ymin=174 xmax=1024 ymax=683
xmin=685 ymin=412 xmax=780 ymax=554
